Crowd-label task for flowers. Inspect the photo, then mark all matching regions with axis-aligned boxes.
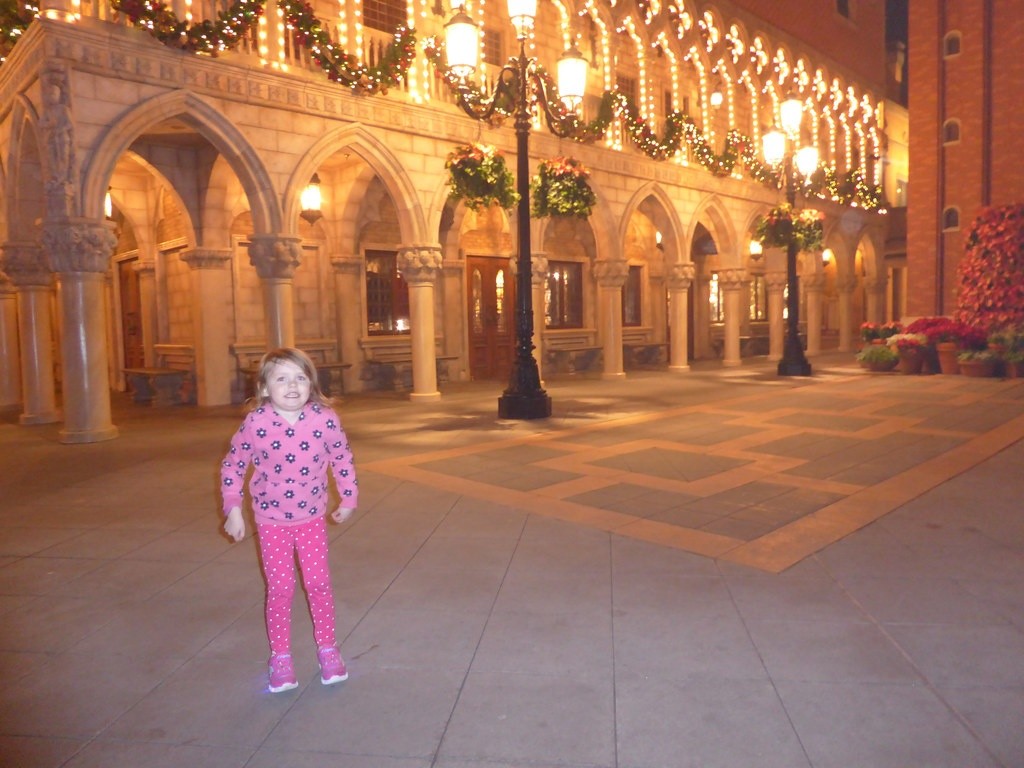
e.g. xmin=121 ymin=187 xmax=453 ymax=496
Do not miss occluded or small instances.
xmin=904 ymin=316 xmax=952 ymax=334
xmin=884 ymin=332 xmax=930 ymax=352
xmin=859 ymin=320 xmax=902 ymax=338
xmin=925 ymin=324 xmax=987 ymax=346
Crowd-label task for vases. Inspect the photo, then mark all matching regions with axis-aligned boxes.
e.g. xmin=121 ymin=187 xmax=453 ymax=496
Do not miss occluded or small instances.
xmin=900 ymin=350 xmax=922 ymax=375
xmin=871 ymin=338 xmax=885 ymax=345
xmin=934 ymin=342 xmax=972 ymax=375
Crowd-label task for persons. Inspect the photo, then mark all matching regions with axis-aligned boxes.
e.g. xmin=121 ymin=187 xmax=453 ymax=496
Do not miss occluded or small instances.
xmin=219 ymin=347 xmax=359 ymax=693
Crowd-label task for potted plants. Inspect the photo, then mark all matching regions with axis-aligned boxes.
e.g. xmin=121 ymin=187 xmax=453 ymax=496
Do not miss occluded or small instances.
xmin=855 ymin=345 xmax=899 ymax=372
xmin=986 ymin=331 xmax=1008 ymax=354
xmin=1003 ymin=353 xmax=1024 ymax=378
xmin=956 ymin=351 xmax=999 ymax=377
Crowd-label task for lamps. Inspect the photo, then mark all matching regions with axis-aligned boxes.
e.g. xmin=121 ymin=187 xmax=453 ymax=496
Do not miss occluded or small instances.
xmin=655 ymin=230 xmax=664 ymax=253
xmin=105 ymin=185 xmax=114 ymax=221
xmin=299 ymin=172 xmax=325 ymax=228
xmin=822 ymin=248 xmax=832 ymax=267
xmin=748 ymin=236 xmax=764 ymax=262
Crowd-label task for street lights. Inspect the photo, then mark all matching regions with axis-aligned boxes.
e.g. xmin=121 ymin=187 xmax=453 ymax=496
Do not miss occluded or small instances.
xmin=762 ymin=86 xmax=820 ymax=375
xmin=443 ymin=0 xmax=590 ymax=419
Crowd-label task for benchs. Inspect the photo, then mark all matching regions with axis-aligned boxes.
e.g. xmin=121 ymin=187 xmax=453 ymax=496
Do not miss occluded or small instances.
xmin=716 ymin=336 xmax=753 ymax=358
xmin=367 ymin=354 xmax=459 ymax=392
xmin=118 ymin=365 xmax=191 ymax=408
xmin=549 ymin=345 xmax=604 ymax=372
xmin=623 ymin=341 xmax=671 ymax=364
xmin=235 ymin=360 xmax=354 ymax=399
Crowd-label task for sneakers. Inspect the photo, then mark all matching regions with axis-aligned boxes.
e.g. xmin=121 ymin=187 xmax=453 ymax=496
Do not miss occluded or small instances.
xmin=267 ymin=650 xmax=299 ymax=693
xmin=316 ymin=641 xmax=348 ymax=685
